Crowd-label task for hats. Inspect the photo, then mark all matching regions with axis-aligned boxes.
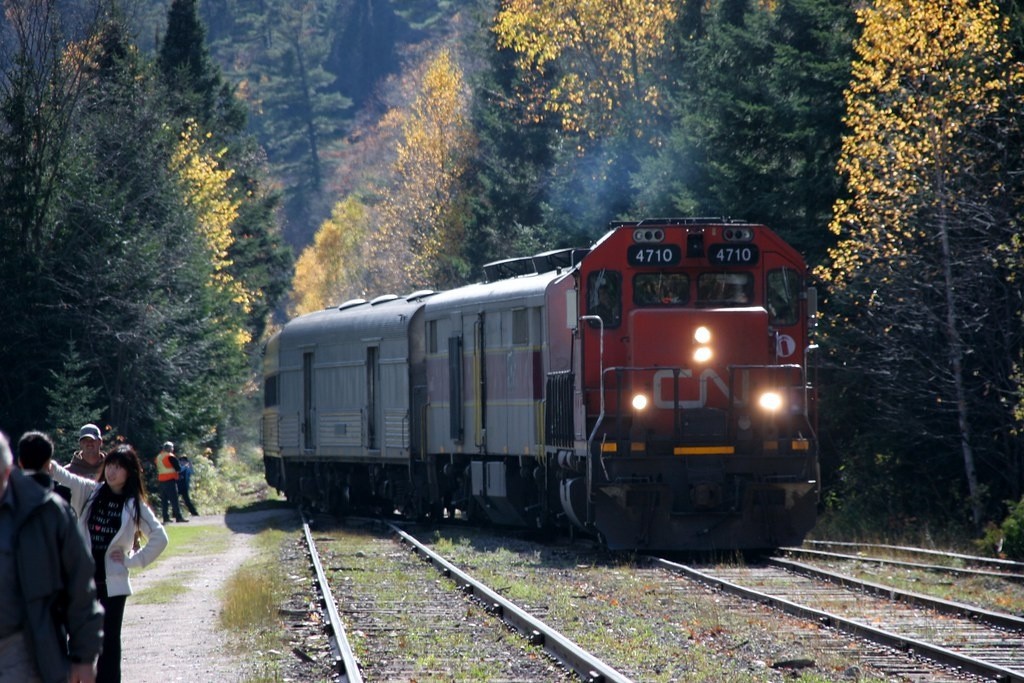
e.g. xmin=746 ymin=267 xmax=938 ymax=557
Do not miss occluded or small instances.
xmin=204 ymin=447 xmax=212 ymax=453
xmin=79 ymin=423 xmax=101 ymax=440
xmin=164 ymin=441 xmax=174 ymax=450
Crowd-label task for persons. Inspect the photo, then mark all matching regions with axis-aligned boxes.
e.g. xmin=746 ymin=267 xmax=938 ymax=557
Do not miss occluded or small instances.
xmin=45 ymin=443 xmax=168 ymax=683
xmin=153 ymin=441 xmax=188 ymax=522
xmin=17 ymin=431 xmax=72 ymax=503
xmin=0 ymin=429 xmax=106 ymax=683
xmin=590 ymin=274 xmax=671 ymax=326
xmin=63 ymin=423 xmax=107 ymax=482
xmin=177 ymin=453 xmax=198 ymax=515
xmin=700 ymin=275 xmax=724 ymax=307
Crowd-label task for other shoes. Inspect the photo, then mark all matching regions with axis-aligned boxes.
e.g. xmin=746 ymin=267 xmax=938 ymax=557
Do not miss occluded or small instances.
xmin=176 ymin=518 xmax=189 ymax=522
xmin=164 ymin=518 xmax=173 ymax=523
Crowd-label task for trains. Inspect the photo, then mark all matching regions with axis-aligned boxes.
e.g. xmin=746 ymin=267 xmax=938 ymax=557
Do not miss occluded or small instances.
xmin=256 ymin=214 xmax=829 ymax=562
